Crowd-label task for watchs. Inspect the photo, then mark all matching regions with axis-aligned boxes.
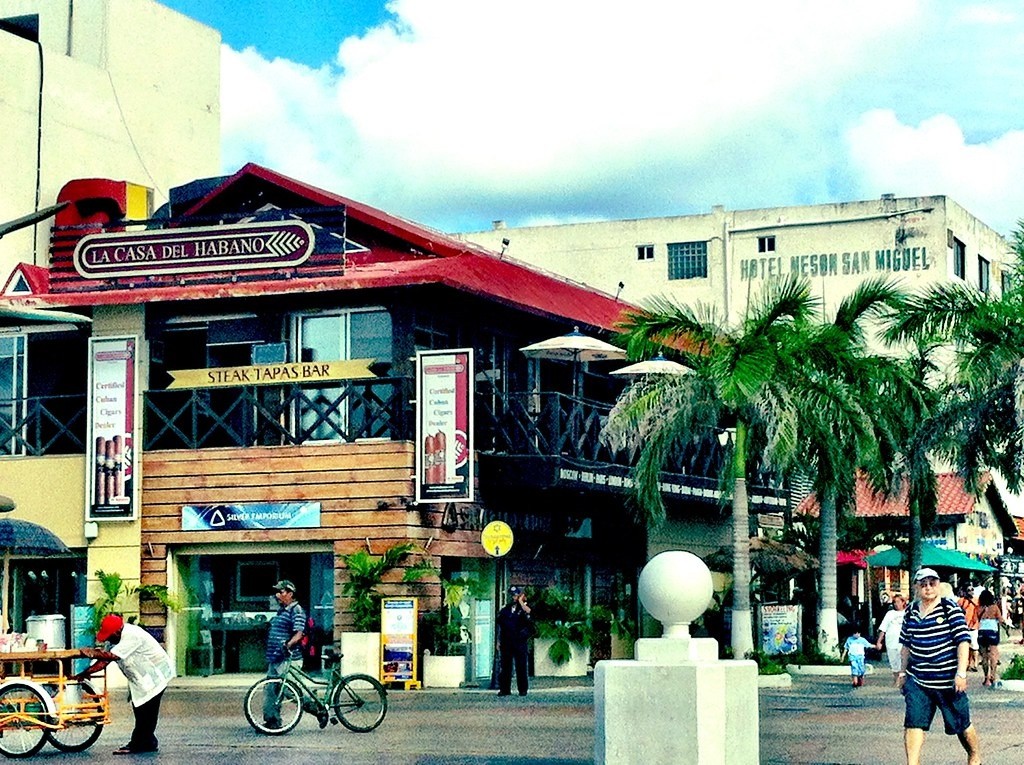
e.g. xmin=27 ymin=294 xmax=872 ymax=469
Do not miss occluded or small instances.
xmin=957 ymin=673 xmax=966 ymax=678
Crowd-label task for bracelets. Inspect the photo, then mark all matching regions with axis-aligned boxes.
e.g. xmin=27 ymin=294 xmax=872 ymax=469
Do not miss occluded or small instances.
xmin=899 ymin=673 xmax=905 ymax=677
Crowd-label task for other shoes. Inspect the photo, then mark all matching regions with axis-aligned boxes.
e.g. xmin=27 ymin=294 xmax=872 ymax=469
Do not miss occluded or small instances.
xmin=256 ymin=723 xmax=283 ymax=734
xmin=991 ymin=675 xmax=996 ymax=681
xmin=967 ymin=665 xmax=977 ymax=671
xmin=518 ymin=691 xmax=527 ymax=696
xmin=317 ymin=714 xmax=328 ymax=729
xmin=852 ymin=678 xmax=864 ymax=688
xmin=497 ymin=691 xmax=511 ymax=696
xmin=113 ymin=745 xmax=159 ymax=755
xmin=982 ymin=679 xmax=992 ymax=686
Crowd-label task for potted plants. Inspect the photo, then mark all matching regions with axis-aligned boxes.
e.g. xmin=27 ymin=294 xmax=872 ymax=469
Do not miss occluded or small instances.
xmin=78 ymin=569 xmax=182 ymax=689
xmin=523 ymin=585 xmax=607 ymax=676
xmin=401 ymin=560 xmax=480 ymax=688
xmin=338 ymin=534 xmax=429 ymax=690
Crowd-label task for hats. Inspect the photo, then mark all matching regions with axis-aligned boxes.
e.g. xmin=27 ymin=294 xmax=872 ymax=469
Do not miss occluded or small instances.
xmin=914 ymin=568 xmax=940 ymax=583
xmin=509 ymin=587 xmax=519 ymax=595
xmin=97 ymin=614 xmax=122 ymax=642
xmin=272 ymin=580 xmax=297 ymax=592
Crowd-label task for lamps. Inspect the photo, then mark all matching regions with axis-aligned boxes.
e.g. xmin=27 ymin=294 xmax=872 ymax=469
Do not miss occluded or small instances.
xmin=615 ymin=282 xmax=624 ymax=299
xmin=500 ymin=238 xmax=509 ymax=260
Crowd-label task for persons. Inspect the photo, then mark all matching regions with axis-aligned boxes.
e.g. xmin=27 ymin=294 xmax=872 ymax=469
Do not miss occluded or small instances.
xmin=262 ymin=580 xmax=329 ymax=729
xmin=74 ymin=615 xmax=177 ymax=755
xmin=957 ymin=578 xmax=1004 ymax=687
xmin=841 ymin=627 xmax=877 ymax=686
xmin=876 ymin=594 xmax=907 ymax=688
xmin=496 ymin=585 xmax=533 ymax=696
xmin=896 ymin=568 xmax=982 ymax=765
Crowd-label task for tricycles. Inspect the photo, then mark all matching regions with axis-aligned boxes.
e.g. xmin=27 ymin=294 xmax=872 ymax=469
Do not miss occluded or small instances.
xmin=0 ymin=638 xmax=113 ymax=759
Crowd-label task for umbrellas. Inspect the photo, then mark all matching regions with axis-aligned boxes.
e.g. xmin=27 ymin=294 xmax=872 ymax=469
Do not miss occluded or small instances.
xmin=519 ymin=325 xmax=627 ymax=439
xmin=609 ymin=351 xmax=693 ymax=378
xmin=865 ymin=541 xmax=998 ymax=572
xmin=703 ymin=536 xmax=819 ymax=571
xmin=0 ymin=518 xmax=73 ymax=634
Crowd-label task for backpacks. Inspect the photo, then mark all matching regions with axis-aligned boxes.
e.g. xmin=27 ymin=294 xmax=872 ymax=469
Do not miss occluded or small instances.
xmin=288 ymin=604 xmax=316 ymax=658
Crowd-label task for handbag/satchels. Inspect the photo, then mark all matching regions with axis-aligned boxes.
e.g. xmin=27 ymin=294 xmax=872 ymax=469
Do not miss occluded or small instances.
xmin=523 ymin=615 xmax=539 ymax=638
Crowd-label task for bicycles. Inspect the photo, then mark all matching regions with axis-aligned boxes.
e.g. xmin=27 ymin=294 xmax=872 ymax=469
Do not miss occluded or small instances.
xmin=243 ymin=642 xmax=389 ymax=736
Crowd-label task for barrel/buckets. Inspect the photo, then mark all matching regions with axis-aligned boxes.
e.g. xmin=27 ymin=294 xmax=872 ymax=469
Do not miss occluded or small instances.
xmin=52 ymin=682 xmax=83 ymax=714
xmin=26 ymin=614 xmax=66 ymax=650
